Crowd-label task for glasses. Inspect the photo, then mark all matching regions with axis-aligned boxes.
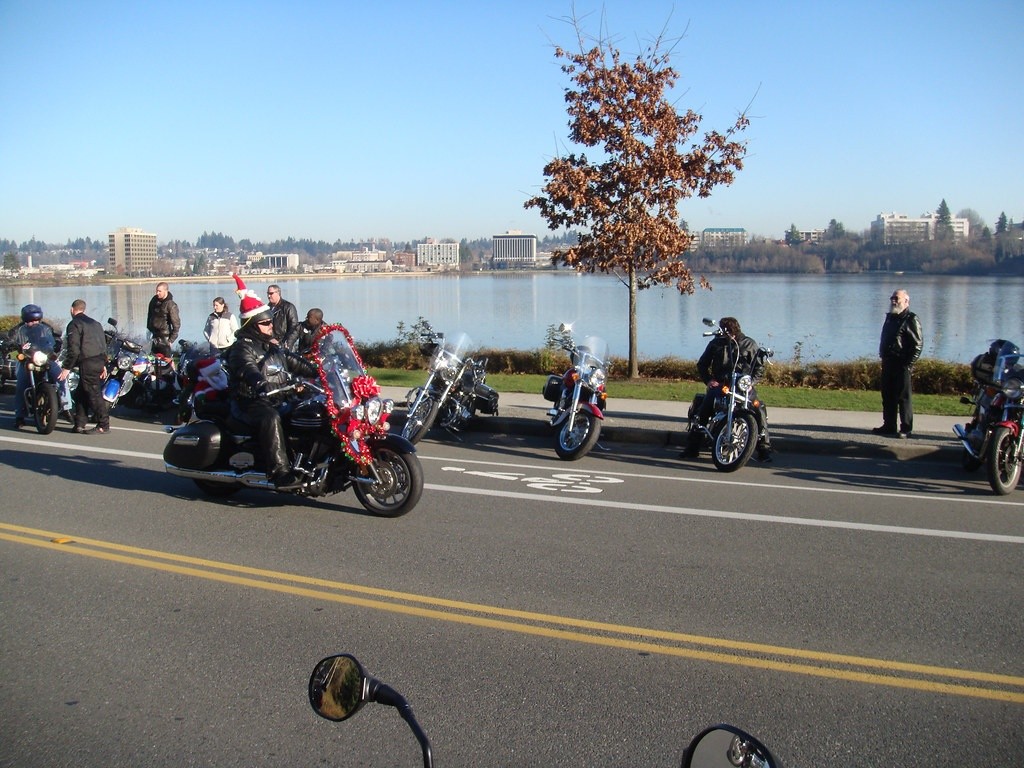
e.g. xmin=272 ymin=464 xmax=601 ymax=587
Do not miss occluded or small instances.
xmin=267 ymin=291 xmax=278 ymax=295
xmin=889 ymin=295 xmax=899 ymax=301
xmin=259 ymin=319 xmax=273 ymax=326
xmin=27 ymin=318 xmax=40 ymax=322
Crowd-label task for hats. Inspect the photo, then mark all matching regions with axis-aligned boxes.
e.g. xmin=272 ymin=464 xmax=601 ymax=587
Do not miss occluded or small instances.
xmin=232 ymin=273 xmax=270 ymax=318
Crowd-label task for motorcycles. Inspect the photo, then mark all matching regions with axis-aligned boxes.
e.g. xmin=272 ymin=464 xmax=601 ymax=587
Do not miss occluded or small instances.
xmin=401 ymin=320 xmax=500 ymax=446
xmin=1 ymin=322 xmax=424 ymax=518
xmin=684 ymin=318 xmax=775 ymax=474
xmin=949 ymin=338 xmax=1024 ymax=497
xmin=541 ymin=334 xmax=612 ymax=462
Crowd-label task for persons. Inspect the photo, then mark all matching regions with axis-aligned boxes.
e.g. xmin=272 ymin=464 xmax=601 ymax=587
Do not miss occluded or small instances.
xmin=147 ymin=282 xmax=181 ymax=357
xmin=203 ymin=297 xmax=239 ymax=359
xmin=679 ymin=317 xmax=773 ymax=463
xmin=57 ymin=299 xmax=111 ymax=434
xmin=873 ymin=289 xmax=924 ymax=438
xmin=267 ymin=285 xmax=334 ymax=350
xmin=2 ymin=304 xmax=75 ymax=429
xmin=224 ymin=275 xmax=349 ymax=486
xmin=193 ymin=357 xmax=228 ymax=406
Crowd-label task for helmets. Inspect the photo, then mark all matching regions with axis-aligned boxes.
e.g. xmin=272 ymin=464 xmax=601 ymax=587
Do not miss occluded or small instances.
xmin=240 ymin=311 xmax=274 ymax=339
xmin=21 ymin=303 xmax=43 ymax=323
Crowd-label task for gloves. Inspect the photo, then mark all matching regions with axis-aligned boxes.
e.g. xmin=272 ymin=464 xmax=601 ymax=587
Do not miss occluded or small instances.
xmin=257 ymin=380 xmax=282 ymax=399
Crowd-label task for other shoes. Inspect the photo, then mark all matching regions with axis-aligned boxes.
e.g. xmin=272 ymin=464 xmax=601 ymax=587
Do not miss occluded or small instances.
xmin=85 ymin=425 xmax=110 ymax=434
xmin=62 ymin=411 xmax=72 ymax=422
xmin=71 ymin=425 xmax=85 ymax=432
xmin=873 ymin=425 xmax=898 ymax=433
xmin=678 ymin=451 xmax=699 ymax=459
xmin=759 ymin=450 xmax=773 ymax=462
xmin=274 ymin=471 xmax=303 ymax=490
xmin=14 ymin=418 xmax=25 ymax=428
xmin=897 ymin=431 xmax=912 ymax=438
